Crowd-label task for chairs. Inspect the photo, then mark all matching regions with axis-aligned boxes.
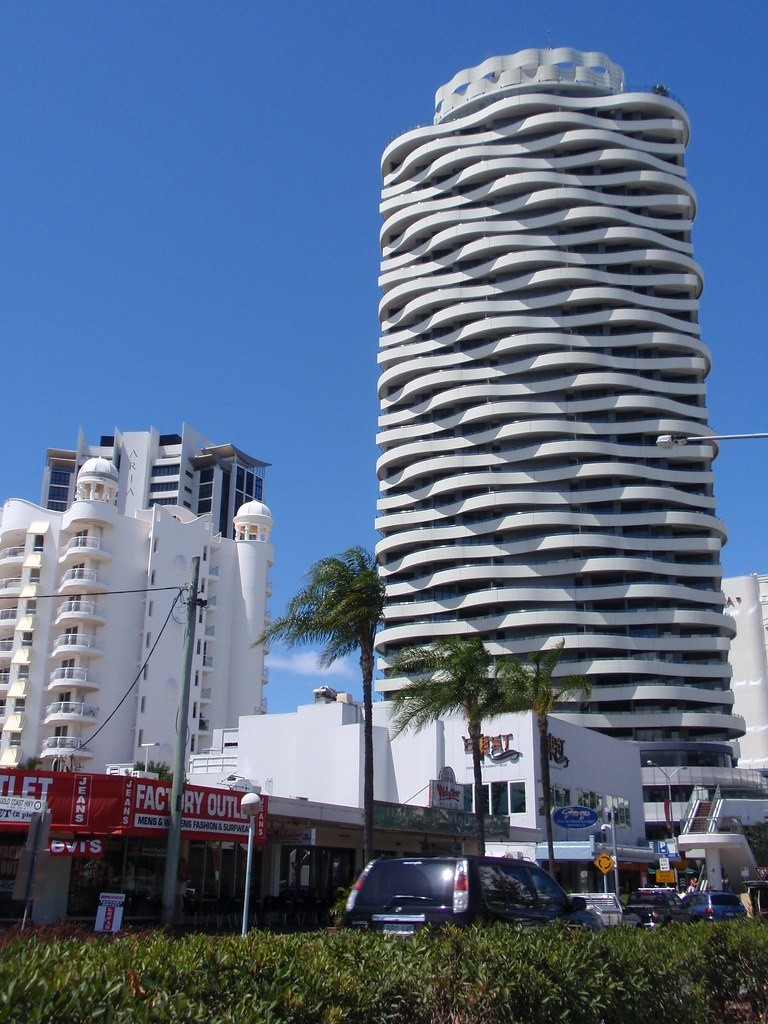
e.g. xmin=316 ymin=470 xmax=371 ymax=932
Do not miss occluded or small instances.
xmin=181 ymin=893 xmax=336 ymax=931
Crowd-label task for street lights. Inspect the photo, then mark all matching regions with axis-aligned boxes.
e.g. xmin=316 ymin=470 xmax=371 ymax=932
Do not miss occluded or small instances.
xmin=239 ymin=792 xmax=264 ymax=936
xmin=647 ymin=760 xmax=687 ymax=896
xmin=600 ymin=805 xmax=620 ymax=899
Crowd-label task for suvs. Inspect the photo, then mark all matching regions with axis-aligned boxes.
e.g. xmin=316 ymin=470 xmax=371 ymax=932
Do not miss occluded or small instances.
xmin=678 ymin=888 xmax=748 ymax=923
xmin=340 ymin=852 xmax=605 ymax=943
xmin=621 ymin=886 xmax=692 ymax=928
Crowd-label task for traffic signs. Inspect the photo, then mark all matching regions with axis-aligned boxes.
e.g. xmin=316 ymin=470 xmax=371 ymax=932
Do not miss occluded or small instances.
xmin=658 ymin=857 xmax=670 ymax=872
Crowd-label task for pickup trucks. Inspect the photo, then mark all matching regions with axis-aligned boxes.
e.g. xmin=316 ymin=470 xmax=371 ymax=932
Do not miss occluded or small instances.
xmin=564 ymin=891 xmax=642 ymax=932
